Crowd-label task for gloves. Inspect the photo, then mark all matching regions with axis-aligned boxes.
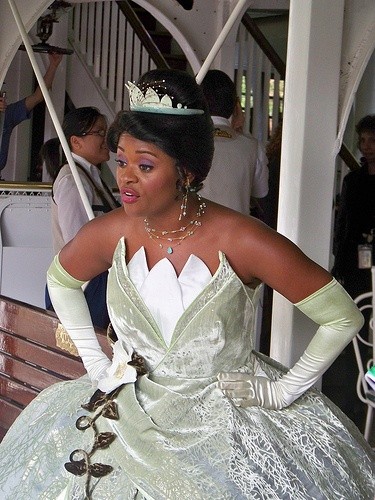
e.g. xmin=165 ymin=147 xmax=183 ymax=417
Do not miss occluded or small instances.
xmin=215 ymin=277 xmax=364 ymax=409
xmin=46 ymin=250 xmax=112 ymax=385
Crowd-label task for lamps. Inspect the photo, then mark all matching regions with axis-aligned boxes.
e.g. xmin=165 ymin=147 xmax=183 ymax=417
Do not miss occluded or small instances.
xmin=17 ymin=0 xmax=75 ymax=57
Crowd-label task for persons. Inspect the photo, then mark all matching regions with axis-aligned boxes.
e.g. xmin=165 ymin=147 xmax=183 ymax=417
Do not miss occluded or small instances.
xmin=0 ymin=67 xmax=375 ymax=500
xmin=334 ymin=114 xmax=375 ymax=418
xmin=0 ymin=54 xmax=64 ymax=172
xmin=44 ymin=105 xmax=109 ymax=324
xmin=197 ymin=70 xmax=269 ymax=217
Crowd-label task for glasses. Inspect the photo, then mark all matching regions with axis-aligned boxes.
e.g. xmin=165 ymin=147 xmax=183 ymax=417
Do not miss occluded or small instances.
xmin=82 ymin=128 xmax=106 ymax=137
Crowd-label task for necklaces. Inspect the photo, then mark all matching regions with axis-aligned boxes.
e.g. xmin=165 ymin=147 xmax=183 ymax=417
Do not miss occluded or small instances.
xmin=143 ymin=193 xmax=208 ymax=255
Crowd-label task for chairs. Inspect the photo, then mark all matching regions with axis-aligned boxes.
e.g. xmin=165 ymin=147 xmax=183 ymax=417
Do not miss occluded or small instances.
xmin=349 ymin=290 xmax=375 ymax=445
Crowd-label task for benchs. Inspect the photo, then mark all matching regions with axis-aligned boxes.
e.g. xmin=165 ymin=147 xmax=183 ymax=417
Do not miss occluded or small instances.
xmin=0 ymin=295 xmax=133 ymax=471
